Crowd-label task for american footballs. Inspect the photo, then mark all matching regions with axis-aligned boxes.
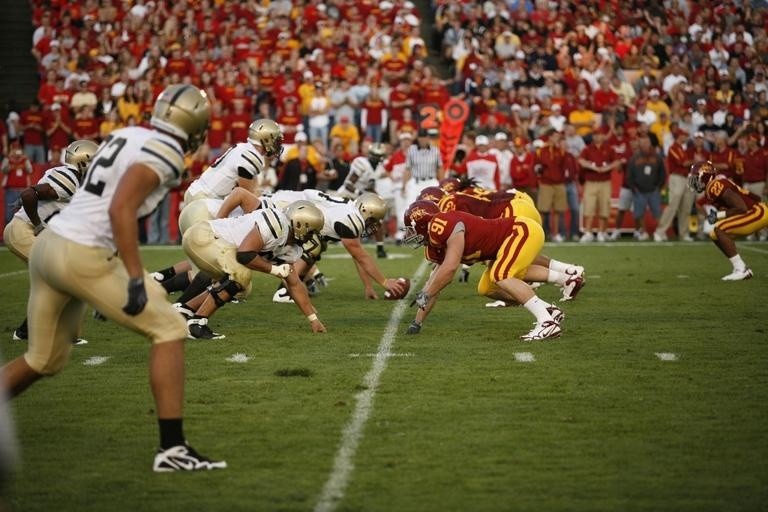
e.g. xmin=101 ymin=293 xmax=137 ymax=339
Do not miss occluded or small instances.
xmin=384 ymin=277 xmax=410 ymax=299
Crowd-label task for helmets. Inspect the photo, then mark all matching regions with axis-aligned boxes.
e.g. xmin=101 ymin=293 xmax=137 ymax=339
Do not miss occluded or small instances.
xmin=366 ymin=142 xmax=386 ymax=163
xmin=65 ymin=139 xmax=99 ymax=178
xmin=151 ymin=84 xmax=211 ymax=154
xmin=687 ymin=161 xmax=717 ymax=193
xmin=248 ymin=118 xmax=284 ymax=161
xmin=283 ymin=201 xmax=324 ymax=245
xmin=355 ymin=192 xmax=388 ymax=237
xmin=399 ymin=178 xmax=461 ymax=249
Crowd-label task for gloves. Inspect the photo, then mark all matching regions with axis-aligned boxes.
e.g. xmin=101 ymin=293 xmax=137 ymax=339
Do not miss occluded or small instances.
xmin=314 ymin=273 xmax=328 ymax=286
xmin=93 ymin=309 xmax=106 ymax=321
xmin=409 ymin=291 xmax=431 ymax=311
xmin=458 ymin=268 xmax=469 ymax=282
xmin=404 ymin=320 xmax=422 ymax=336
xmin=270 ymin=263 xmax=295 ymax=279
xmin=306 ymin=279 xmax=321 ymax=297
xmin=122 ymin=278 xmax=147 ymax=316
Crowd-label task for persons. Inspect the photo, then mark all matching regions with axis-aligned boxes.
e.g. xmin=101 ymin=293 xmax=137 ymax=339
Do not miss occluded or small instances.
xmin=0 ymin=84 xmax=586 ymax=473
xmin=0 ymin=1 xmax=768 ymax=249
xmin=687 ymin=160 xmax=768 ymax=282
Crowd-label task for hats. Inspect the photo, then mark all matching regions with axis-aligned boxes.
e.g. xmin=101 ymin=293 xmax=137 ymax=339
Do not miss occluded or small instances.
xmin=592 ymin=127 xmax=602 ymax=134
xmin=475 ymin=132 xmax=549 ymax=147
xmin=295 ymin=131 xmax=308 ymax=142
xmin=398 ymin=127 xmax=438 ymax=140
xmin=673 ymin=127 xmax=728 ymax=141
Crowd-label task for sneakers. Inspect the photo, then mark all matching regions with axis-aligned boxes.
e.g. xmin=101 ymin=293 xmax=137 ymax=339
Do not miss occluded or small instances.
xmin=721 ymin=265 xmax=754 ymax=280
xmin=272 ymin=286 xmax=297 ymax=303
xmin=519 ymin=305 xmax=565 ymax=342
xmin=552 ymin=229 xmax=694 ymax=242
xmin=154 ymin=436 xmax=228 ymax=473
xmin=485 ymin=281 xmax=545 ymax=308
xmin=559 ymin=265 xmax=586 ymax=303
xmin=172 ymin=302 xmax=224 ymax=340
xmin=12 ymin=328 xmax=89 ymax=345
